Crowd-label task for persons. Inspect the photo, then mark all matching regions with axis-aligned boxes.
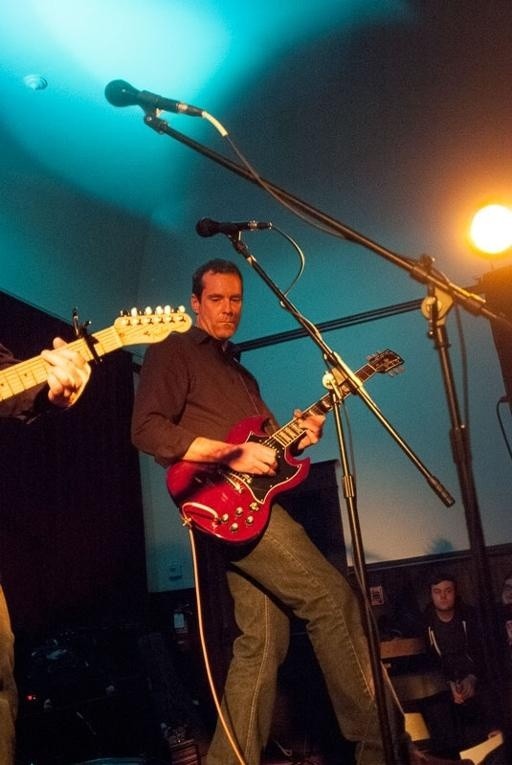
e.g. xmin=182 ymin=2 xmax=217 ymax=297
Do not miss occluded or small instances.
xmin=1 ymin=331 xmax=95 ymax=765
xmin=423 ymin=564 xmax=492 ymax=753
xmin=128 ymin=256 xmax=476 ymax=765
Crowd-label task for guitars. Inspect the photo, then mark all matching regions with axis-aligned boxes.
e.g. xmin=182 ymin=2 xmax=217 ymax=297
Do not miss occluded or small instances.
xmin=1 ymin=305 xmax=193 ymax=401
xmin=165 ymin=348 xmax=406 ymax=546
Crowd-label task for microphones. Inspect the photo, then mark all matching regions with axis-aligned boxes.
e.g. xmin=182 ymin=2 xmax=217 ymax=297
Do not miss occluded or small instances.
xmin=195 ymin=216 xmax=273 ymax=238
xmin=104 ymin=78 xmax=204 ymax=117
